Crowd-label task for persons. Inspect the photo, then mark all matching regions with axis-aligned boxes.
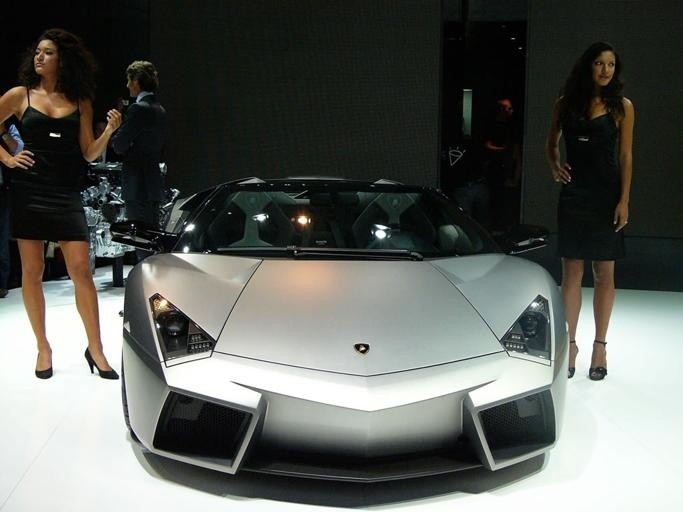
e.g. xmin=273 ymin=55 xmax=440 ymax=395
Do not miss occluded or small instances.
xmin=0 ymin=122 xmax=25 ymax=298
xmin=0 ymin=27 xmax=123 ymax=380
xmin=479 ymin=97 xmax=523 ymax=231
xmin=545 ymin=42 xmax=635 ymax=382
xmin=108 ymin=60 xmax=169 ymax=263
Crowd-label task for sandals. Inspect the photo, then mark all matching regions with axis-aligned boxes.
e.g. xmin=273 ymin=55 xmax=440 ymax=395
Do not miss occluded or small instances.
xmin=589 ymin=340 xmax=606 ymax=380
xmin=568 ymin=340 xmax=577 ymax=378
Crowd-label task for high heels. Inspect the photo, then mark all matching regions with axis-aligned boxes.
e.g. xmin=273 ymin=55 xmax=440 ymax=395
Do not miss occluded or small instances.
xmin=84 ymin=347 xmax=120 ymax=379
xmin=35 ymin=349 xmax=53 ymax=379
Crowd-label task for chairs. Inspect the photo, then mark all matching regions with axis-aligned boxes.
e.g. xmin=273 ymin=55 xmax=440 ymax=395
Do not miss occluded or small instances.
xmin=201 ymin=174 xmax=439 ymax=256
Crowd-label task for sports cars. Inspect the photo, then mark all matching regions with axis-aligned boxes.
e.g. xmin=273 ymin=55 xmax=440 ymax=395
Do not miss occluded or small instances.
xmin=110 ymin=176 xmax=571 ymax=484
